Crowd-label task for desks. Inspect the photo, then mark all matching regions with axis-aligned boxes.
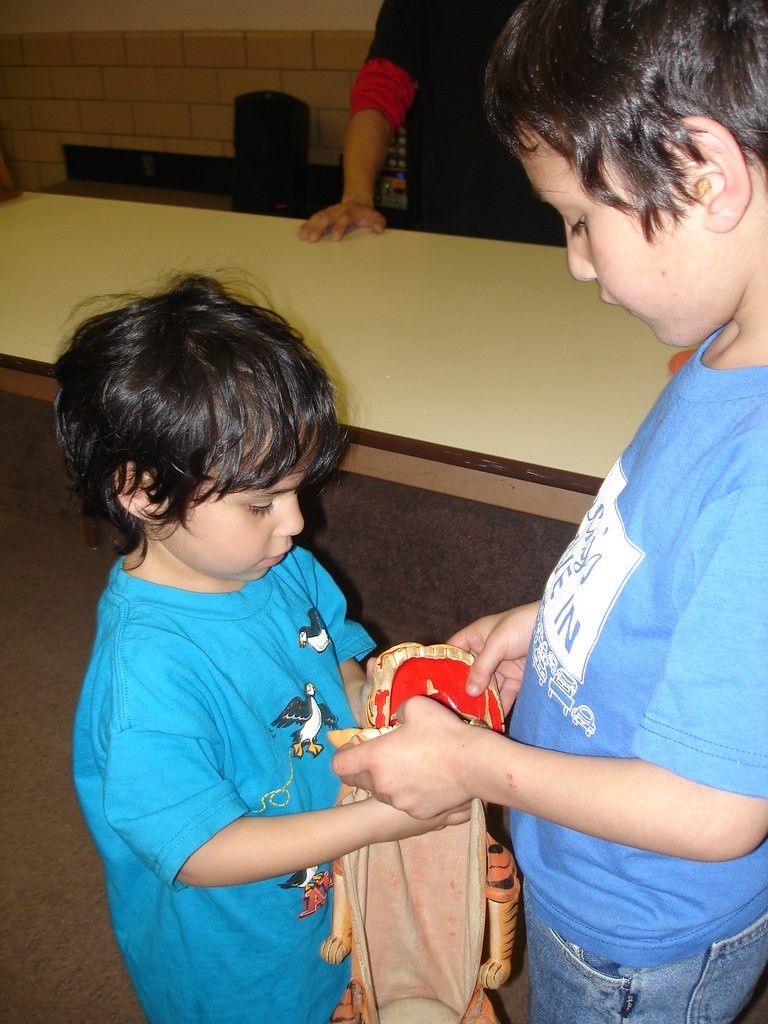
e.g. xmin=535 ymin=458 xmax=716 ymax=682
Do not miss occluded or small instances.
xmin=1 ymin=190 xmax=705 ymax=523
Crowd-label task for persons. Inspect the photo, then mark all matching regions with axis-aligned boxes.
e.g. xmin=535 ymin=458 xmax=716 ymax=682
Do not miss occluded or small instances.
xmin=54 ymin=270 xmax=473 ymax=1023
xmin=301 ymin=0 xmax=768 ymax=1024
